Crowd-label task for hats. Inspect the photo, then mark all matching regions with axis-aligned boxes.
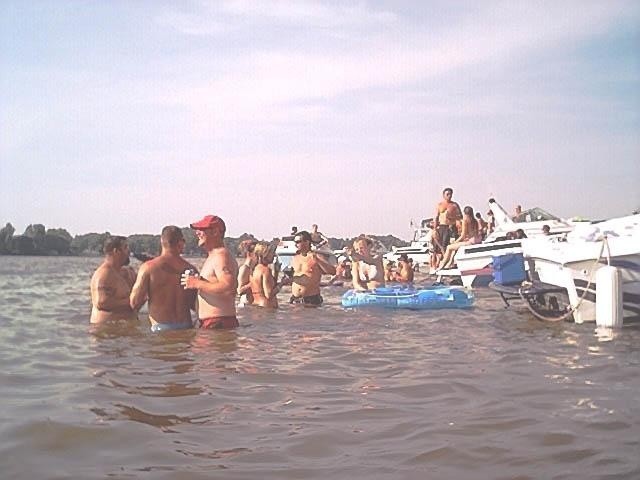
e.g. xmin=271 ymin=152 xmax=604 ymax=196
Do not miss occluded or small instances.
xmin=190 ymin=214 xmax=226 ymax=234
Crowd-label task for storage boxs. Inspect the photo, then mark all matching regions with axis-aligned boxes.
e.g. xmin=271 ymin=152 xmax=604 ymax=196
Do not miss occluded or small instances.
xmin=491 ymin=252 xmax=525 ymax=285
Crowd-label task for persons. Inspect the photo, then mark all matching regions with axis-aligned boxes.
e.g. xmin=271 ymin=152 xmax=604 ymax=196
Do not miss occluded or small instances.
xmin=431 ymin=188 xmax=495 ymax=269
xmin=285 ymin=230 xmax=341 ymax=312
xmin=89 ymin=234 xmax=141 ymax=331
xmin=131 ymin=221 xmax=201 ymax=337
xmin=259 ymin=225 xmax=414 ymax=292
xmin=251 ymin=238 xmax=294 ymax=318
xmin=506 ymin=205 xmax=550 ymax=239
xmin=229 ymin=234 xmax=259 ymax=310
xmin=181 ymin=213 xmax=246 ymax=332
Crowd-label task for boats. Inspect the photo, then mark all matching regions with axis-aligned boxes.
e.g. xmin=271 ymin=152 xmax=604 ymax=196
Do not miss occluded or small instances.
xmin=341 ymin=286 xmax=475 ymax=312
xmin=387 ymin=196 xmax=639 ymax=327
xmin=272 ymin=236 xmax=338 ymax=275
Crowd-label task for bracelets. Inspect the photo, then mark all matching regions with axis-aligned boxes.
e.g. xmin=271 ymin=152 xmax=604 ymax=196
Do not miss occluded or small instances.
xmin=276 ymin=284 xmax=281 ymax=292
